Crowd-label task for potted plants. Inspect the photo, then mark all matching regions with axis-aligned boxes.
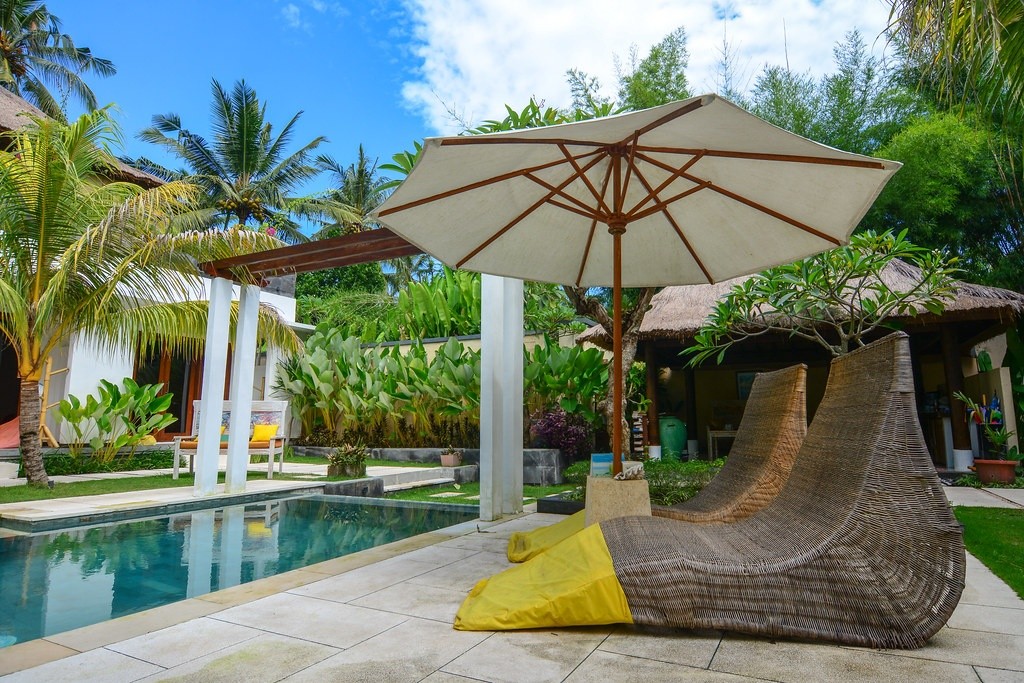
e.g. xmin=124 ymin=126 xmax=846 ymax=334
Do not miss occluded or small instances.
xmin=323 ymin=438 xmax=368 ymax=478
xmin=952 ymin=389 xmax=1024 ymax=485
xmin=441 ymin=445 xmax=463 ymax=466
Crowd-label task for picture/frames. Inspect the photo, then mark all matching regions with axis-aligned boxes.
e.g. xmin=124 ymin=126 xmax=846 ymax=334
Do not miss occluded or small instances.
xmin=736 ymin=369 xmax=764 ymax=400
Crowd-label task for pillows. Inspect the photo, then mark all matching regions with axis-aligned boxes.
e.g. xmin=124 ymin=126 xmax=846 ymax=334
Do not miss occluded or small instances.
xmin=193 ymin=426 xmax=228 ymax=441
xmin=251 ymin=424 xmax=279 ymax=441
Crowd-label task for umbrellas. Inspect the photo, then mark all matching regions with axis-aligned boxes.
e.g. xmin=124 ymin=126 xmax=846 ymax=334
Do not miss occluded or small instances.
xmin=368 ymin=95 xmax=905 ymax=476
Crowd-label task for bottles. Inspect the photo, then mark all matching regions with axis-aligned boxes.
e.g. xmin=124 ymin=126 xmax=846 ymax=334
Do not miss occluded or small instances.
xmin=934 ymin=399 xmax=937 ymax=411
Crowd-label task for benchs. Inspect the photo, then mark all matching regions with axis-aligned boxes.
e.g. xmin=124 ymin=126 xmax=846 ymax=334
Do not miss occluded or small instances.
xmin=172 ymin=399 xmax=291 ymax=480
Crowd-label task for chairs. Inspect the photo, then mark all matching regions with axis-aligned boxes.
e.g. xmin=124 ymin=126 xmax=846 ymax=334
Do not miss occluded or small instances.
xmin=508 ymin=362 xmax=808 ymax=566
xmin=451 ymin=327 xmax=966 ymax=651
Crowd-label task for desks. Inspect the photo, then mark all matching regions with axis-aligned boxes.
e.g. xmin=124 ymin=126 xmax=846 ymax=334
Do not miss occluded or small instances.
xmin=706 ymin=430 xmax=738 ymax=461
xmin=976 ymin=423 xmax=1002 ymax=458
